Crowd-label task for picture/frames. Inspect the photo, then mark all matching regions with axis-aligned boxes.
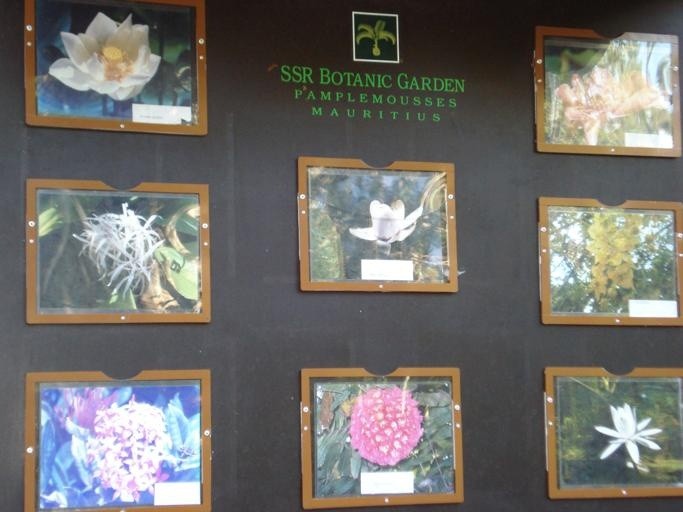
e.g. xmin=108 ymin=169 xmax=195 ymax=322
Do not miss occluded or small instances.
xmin=300 ymin=367 xmax=465 ymax=510
xmin=297 ymin=156 xmax=459 ymax=293
xmin=544 ymin=367 xmax=683 ymax=500
xmin=24 ymin=369 xmax=211 ymax=512
xmin=534 ymin=26 xmax=681 ymax=159
xmin=26 ymin=178 xmax=211 ymax=325
xmin=24 ymin=0 xmax=208 ymax=136
xmin=538 ymin=196 xmax=683 ymax=327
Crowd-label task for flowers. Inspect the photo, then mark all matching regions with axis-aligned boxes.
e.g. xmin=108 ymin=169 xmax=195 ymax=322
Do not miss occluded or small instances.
xmin=71 ymin=202 xmax=170 ymax=302
xmin=554 ymin=66 xmax=663 ymax=128
xmin=52 ymin=386 xmax=178 ymax=502
xmin=348 ymin=200 xmax=424 ymax=255
xmin=587 ymin=212 xmax=666 ymax=309
xmin=47 ymin=12 xmax=161 ymax=101
xmin=594 ymin=403 xmax=662 ymax=464
xmin=348 ymin=386 xmax=423 ymax=466
